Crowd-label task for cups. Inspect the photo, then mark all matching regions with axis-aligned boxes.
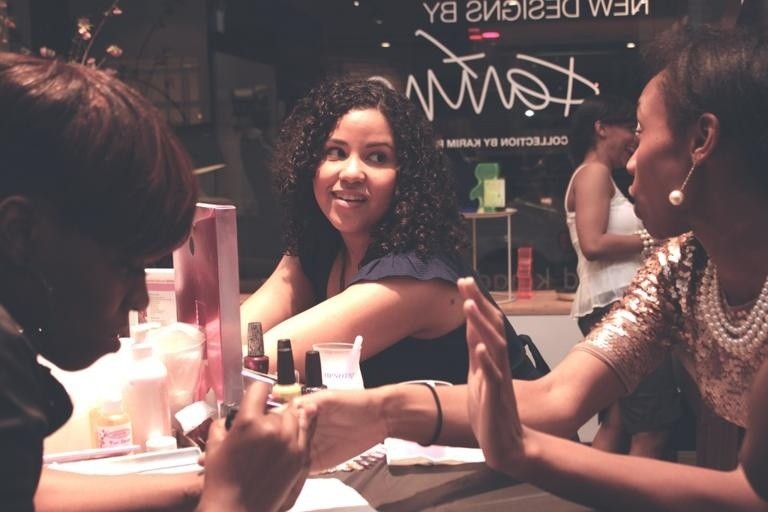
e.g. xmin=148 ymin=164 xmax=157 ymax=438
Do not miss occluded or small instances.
xmin=312 ymin=342 xmax=362 ymax=390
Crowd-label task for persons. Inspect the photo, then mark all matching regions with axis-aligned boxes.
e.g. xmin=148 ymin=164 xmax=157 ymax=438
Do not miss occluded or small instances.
xmin=244 ymin=71 xmax=527 ymax=392
xmin=196 ymin=30 xmax=768 ymax=512
xmin=0 ymin=45 xmax=309 ymax=512
xmin=559 ymin=92 xmax=682 ymax=461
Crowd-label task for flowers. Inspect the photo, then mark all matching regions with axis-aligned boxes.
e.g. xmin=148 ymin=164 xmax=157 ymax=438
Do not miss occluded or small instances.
xmin=0 ymin=1 xmax=124 ymax=78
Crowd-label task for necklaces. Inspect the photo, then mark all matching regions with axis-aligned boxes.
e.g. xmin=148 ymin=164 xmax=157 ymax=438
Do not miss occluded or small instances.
xmin=697 ymin=256 xmax=768 ymax=359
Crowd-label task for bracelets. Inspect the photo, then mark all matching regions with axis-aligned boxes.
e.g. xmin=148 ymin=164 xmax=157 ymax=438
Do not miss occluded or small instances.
xmin=410 ymin=381 xmax=445 ymax=448
xmin=642 ymin=229 xmax=656 ymax=258
xmin=632 ymin=227 xmax=648 ymax=258
